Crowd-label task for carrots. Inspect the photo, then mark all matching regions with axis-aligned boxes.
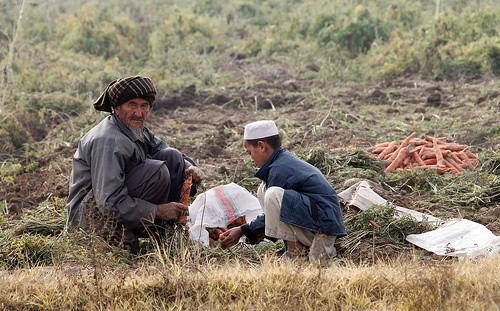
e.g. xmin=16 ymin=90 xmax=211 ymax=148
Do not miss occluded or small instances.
xmin=367 ymin=132 xmax=477 ymax=175
xmin=206 ymin=217 xmax=245 ymax=241
xmin=178 ymin=175 xmax=193 ymax=222
